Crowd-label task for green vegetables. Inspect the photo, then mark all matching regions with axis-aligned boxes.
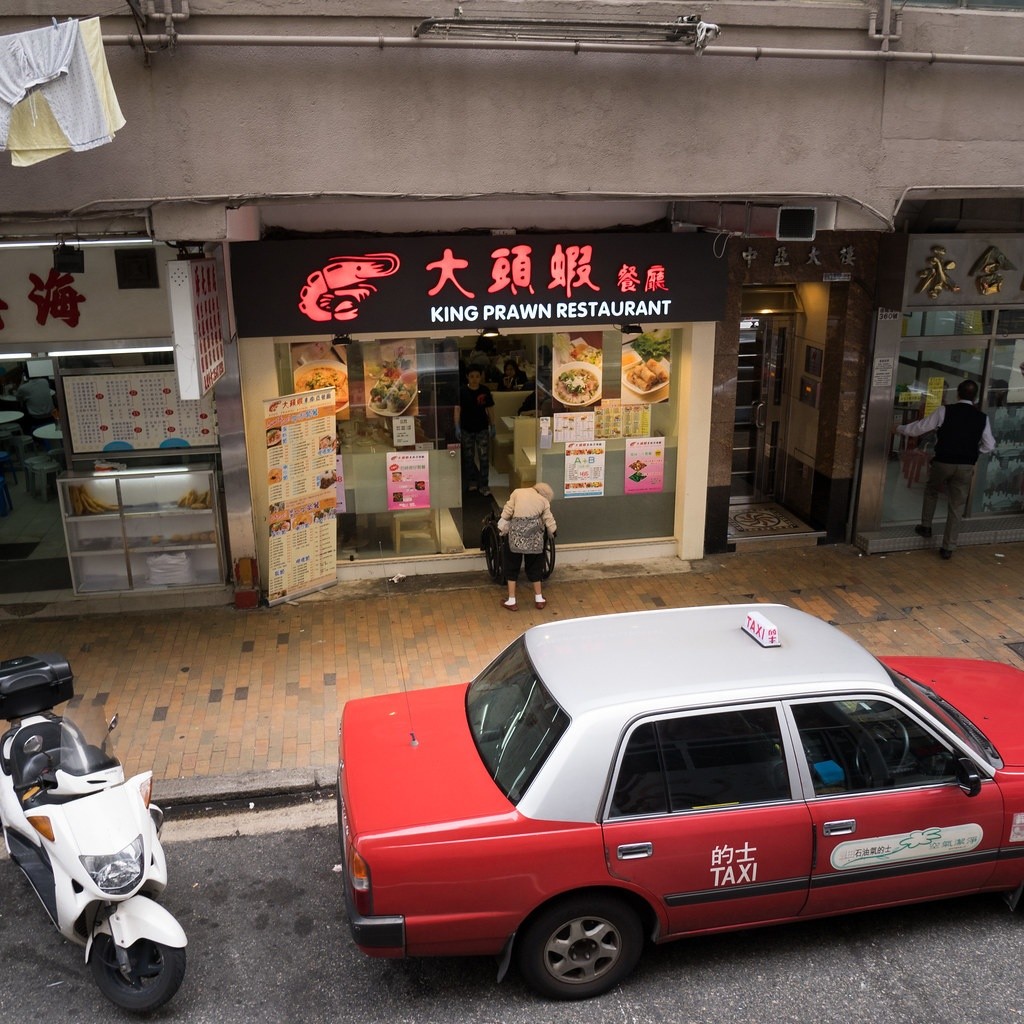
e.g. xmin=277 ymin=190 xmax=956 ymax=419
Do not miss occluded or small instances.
xmin=558 ymin=372 xmax=586 ymax=393
xmin=631 ymin=328 xmax=671 ymax=362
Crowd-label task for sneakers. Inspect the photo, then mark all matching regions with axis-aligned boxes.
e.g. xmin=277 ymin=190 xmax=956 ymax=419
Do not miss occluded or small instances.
xmin=915 ymin=524 xmax=932 ymax=537
xmin=479 ymin=484 xmax=491 ymax=495
xmin=940 ymin=547 xmax=952 ymax=560
xmin=469 ymin=481 xmax=478 ymax=491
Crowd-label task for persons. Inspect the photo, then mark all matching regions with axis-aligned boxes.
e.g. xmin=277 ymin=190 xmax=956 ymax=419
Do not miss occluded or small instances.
xmin=496 ymin=359 xmax=529 ymax=392
xmin=917 ymin=381 xmax=949 ymax=448
xmin=429 ymin=340 xmax=466 ymax=416
xmin=496 ymin=483 xmax=558 ymax=611
xmin=15 ymin=364 xmax=56 ymax=426
xmin=469 ymin=336 xmax=494 ymax=366
xmin=518 ymin=383 xmax=563 ymax=417
xmin=453 ymin=365 xmax=496 ymax=496
xmin=890 ymin=380 xmax=996 ymax=559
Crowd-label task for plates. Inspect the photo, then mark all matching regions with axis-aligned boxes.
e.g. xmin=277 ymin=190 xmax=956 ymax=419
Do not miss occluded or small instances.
xmin=622 ymin=356 xmax=670 ymax=394
xmin=368 ymin=373 xmax=418 ymax=417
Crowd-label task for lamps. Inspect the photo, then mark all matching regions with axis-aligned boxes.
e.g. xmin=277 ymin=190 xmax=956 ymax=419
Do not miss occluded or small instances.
xmin=330 ymin=334 xmax=352 ymax=347
xmin=613 ymin=323 xmax=644 ymax=335
xmin=482 ymin=328 xmax=500 ymax=337
xmin=52 ymin=244 xmax=84 ymax=273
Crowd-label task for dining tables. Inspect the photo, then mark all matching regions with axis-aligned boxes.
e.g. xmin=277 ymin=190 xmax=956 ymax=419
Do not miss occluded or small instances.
xmin=522 ymin=439 xmax=626 ymax=466
xmin=503 ymin=415 xmax=531 ymax=430
xmin=342 ymin=436 xmax=397 ymax=550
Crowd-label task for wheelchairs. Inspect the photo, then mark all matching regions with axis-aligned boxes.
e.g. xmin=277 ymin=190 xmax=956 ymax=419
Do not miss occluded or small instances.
xmin=481 ymin=512 xmax=557 ymax=581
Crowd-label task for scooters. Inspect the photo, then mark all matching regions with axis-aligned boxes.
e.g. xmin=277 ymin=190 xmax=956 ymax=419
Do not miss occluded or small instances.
xmin=1 ymin=655 xmax=189 ymax=1015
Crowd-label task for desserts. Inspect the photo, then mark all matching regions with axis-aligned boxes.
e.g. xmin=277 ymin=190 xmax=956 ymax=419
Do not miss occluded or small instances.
xmin=370 ymin=366 xmax=419 ymax=412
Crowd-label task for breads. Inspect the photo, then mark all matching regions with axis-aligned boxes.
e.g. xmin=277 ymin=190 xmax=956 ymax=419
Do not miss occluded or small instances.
xmin=69 ymin=485 xmax=120 ymax=514
xmin=149 ymin=530 xmax=216 ymax=542
xmin=177 ymin=488 xmax=213 ymax=509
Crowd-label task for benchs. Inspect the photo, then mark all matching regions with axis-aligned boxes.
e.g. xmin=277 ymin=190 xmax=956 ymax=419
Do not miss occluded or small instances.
xmin=491 ymin=389 xmax=554 ymax=491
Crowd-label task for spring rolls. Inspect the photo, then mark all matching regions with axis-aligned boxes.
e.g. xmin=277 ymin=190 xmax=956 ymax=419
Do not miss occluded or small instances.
xmin=628 ymin=357 xmax=669 ymax=391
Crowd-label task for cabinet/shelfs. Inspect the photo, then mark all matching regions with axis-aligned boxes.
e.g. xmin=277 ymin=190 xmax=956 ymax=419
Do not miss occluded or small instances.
xmin=51 ymin=461 xmax=226 ymax=595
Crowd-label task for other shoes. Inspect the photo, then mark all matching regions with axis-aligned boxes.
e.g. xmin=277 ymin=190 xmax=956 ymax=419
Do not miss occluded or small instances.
xmin=536 ymin=598 xmax=546 ymax=609
xmin=500 ymin=599 xmax=518 ymax=611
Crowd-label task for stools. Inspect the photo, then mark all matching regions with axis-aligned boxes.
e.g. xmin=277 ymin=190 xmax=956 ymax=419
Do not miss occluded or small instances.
xmin=0 ymin=424 xmax=64 ymax=518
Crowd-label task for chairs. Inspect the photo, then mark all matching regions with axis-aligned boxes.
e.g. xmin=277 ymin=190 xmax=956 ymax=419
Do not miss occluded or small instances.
xmin=391 ymin=508 xmax=441 ymax=555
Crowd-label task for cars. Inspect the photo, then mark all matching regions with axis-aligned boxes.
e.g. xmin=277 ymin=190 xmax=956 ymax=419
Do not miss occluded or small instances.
xmin=333 ymin=599 xmax=1022 ymax=1003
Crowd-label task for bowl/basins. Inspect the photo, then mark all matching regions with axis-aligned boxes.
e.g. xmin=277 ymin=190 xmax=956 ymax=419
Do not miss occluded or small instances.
xmin=293 ymin=360 xmax=349 ymax=414
xmin=553 ymin=361 xmax=602 ymax=406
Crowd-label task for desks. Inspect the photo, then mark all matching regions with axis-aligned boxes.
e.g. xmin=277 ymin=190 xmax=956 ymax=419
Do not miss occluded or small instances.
xmin=32 ymin=423 xmax=64 ymax=448
xmin=0 ymin=411 xmax=24 ymax=423
xmin=0 ymin=389 xmax=56 ymax=401
